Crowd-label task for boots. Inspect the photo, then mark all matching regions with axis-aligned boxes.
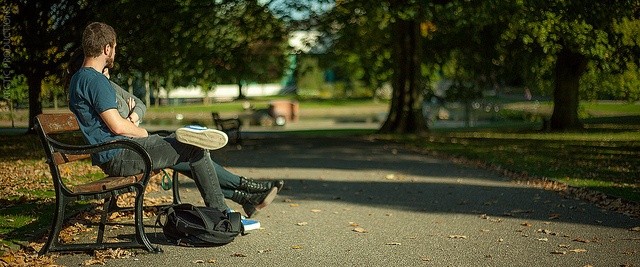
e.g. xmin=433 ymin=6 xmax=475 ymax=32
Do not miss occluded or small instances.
xmin=239 ymin=176 xmax=283 ymax=194
xmin=232 ymin=190 xmax=277 ymax=218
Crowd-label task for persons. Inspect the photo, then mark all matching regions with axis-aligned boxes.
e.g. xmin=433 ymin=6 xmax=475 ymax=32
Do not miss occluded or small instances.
xmin=69 ymin=23 xmax=261 ymax=231
xmin=103 ymin=66 xmax=284 ymax=218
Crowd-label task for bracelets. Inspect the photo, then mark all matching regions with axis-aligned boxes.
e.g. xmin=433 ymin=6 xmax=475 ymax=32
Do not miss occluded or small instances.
xmin=129 ymin=116 xmax=135 ymax=124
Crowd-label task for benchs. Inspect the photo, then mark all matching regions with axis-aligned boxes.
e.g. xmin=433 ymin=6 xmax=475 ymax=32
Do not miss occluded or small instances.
xmin=212 ymin=113 xmax=240 ymax=145
xmin=33 ymin=114 xmax=182 ymax=257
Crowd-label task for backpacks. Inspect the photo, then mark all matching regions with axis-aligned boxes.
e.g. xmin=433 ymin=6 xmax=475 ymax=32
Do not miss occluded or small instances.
xmin=155 ymin=203 xmax=251 ymax=246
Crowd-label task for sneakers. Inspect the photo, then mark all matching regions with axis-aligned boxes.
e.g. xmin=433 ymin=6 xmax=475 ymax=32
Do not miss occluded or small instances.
xmin=227 ymin=209 xmax=260 ymax=231
xmin=177 ymin=125 xmax=228 ymax=150
